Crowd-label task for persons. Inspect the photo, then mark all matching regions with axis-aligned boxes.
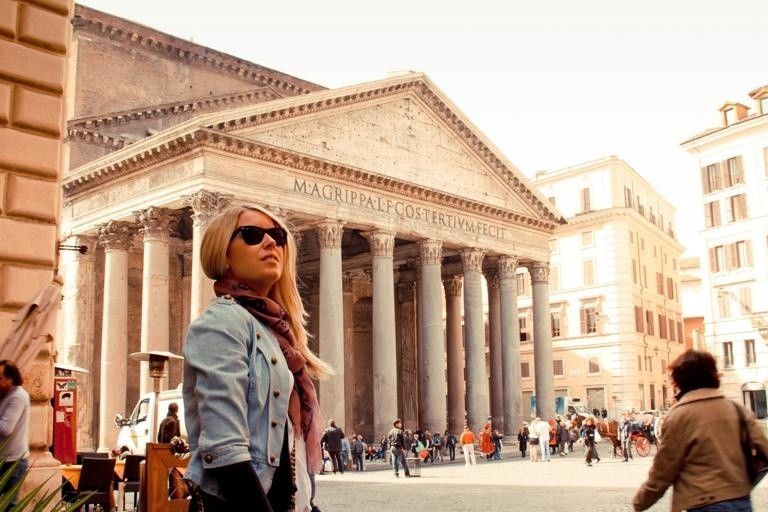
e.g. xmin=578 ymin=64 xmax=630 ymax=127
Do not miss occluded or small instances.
xmin=158 ymin=403 xmax=180 ymax=443
xmin=631 ymin=349 xmax=767 ymax=512
xmin=0 ymin=359 xmax=30 ymax=511
xmin=181 ymin=203 xmax=335 ymax=510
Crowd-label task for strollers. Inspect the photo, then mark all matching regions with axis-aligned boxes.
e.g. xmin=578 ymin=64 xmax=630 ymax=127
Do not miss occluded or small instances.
xmin=365 ymin=446 xmax=384 ymax=462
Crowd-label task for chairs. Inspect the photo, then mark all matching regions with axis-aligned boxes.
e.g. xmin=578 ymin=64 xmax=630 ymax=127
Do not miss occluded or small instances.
xmin=56 ymin=447 xmax=144 ymax=512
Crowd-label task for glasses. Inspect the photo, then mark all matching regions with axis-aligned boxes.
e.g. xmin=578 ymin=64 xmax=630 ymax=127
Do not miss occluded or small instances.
xmin=230 ymin=225 xmax=287 ymax=246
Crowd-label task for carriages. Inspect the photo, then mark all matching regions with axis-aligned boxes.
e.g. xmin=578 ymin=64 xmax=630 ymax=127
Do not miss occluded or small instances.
xmin=594 ymin=408 xmax=661 ymax=457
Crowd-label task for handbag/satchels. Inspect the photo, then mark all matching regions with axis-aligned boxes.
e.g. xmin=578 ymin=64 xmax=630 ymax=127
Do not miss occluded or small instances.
xmin=732 ymin=401 xmax=767 ymax=487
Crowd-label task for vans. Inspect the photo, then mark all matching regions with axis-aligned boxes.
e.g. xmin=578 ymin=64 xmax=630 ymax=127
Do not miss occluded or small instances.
xmin=111 ymin=381 xmax=189 ymax=463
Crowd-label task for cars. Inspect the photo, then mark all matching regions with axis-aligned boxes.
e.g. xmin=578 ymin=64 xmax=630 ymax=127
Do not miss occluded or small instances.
xmin=486 ymin=416 xmax=529 ymax=436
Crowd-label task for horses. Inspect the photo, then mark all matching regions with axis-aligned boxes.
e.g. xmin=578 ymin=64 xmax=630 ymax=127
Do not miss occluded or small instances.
xmin=585 ymin=415 xmax=623 ymax=457
xmin=566 ymin=405 xmax=586 ymax=429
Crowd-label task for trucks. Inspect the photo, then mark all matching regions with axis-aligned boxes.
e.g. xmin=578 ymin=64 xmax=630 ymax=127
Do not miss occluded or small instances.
xmin=528 ymin=394 xmax=595 ymax=420
